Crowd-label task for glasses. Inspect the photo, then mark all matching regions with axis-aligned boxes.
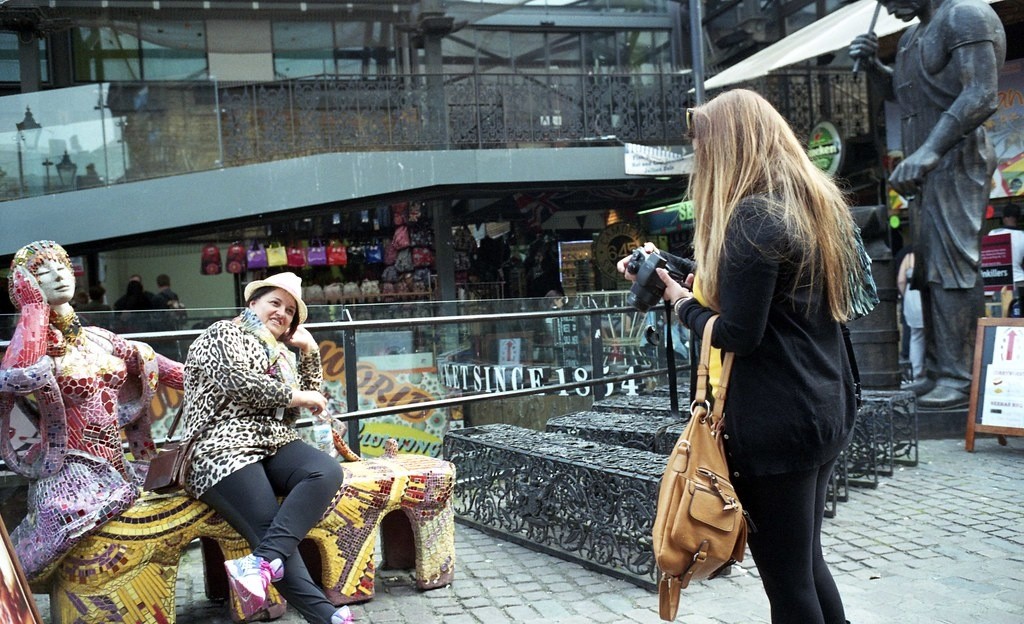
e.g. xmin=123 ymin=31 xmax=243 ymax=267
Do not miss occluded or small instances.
xmin=685 ymin=108 xmax=697 ymax=129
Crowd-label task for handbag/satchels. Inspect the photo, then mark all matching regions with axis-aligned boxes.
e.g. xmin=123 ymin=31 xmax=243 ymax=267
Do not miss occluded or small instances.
xmin=302 ymin=279 xmax=430 ymax=303
xmin=289 ymin=238 xmax=306 ymax=267
xmin=267 ymin=237 xmax=289 ymax=266
xmin=327 ymin=236 xmax=346 ymax=265
xmin=247 ymin=239 xmax=267 ymax=268
xmin=653 ymin=313 xmax=748 ymax=622
xmin=307 ymin=235 xmax=328 ymax=264
xmin=347 ymin=201 xmax=475 ymax=281
xmin=144 ymin=444 xmax=191 ymax=492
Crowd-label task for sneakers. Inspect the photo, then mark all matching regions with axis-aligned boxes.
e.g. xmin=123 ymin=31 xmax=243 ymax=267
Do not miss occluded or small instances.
xmin=224 ymin=555 xmax=286 ymax=619
xmin=331 ymin=605 xmax=355 ymax=624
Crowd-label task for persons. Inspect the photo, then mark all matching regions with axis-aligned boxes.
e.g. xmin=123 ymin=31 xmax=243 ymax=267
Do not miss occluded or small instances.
xmin=181 ymin=273 xmax=359 ymax=624
xmin=849 ymin=1 xmax=1006 ymax=413
xmin=459 ymin=270 xmax=493 ymax=362
xmin=988 ymin=204 xmax=1024 ymax=303
xmin=897 ymin=251 xmax=925 ymax=381
xmin=540 ymin=287 xmax=693 ymax=362
xmin=72 ymin=273 xmax=186 ymax=362
xmin=0 ymin=240 xmax=186 ymax=577
xmin=615 ymin=90 xmax=880 ymax=624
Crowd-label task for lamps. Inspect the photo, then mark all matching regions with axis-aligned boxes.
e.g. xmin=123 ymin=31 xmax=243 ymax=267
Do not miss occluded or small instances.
xmin=56 ymin=150 xmax=77 ymax=191
xmin=15 ymin=105 xmax=43 ymax=197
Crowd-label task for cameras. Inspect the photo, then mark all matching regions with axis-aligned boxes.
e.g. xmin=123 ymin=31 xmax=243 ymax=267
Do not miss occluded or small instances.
xmin=625 ymin=246 xmax=684 ymax=314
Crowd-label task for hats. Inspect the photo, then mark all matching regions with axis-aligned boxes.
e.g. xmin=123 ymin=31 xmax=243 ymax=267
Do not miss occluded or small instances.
xmin=246 ymin=273 xmax=308 ymax=322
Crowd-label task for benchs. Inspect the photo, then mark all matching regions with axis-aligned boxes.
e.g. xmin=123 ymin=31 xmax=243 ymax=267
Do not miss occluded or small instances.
xmin=48 ymin=453 xmax=460 ymax=624
xmin=443 ymin=383 xmax=920 ymax=592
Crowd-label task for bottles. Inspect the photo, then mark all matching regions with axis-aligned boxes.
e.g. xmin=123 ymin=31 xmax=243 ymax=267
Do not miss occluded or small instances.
xmin=312 ymin=405 xmax=335 ymax=456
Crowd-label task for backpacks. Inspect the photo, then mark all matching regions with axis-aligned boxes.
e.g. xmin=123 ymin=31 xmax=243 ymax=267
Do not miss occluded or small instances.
xmin=226 ymin=242 xmax=246 ymax=273
xmin=203 ymin=246 xmax=224 ymax=274
xmin=159 ymin=292 xmax=187 ymax=325
